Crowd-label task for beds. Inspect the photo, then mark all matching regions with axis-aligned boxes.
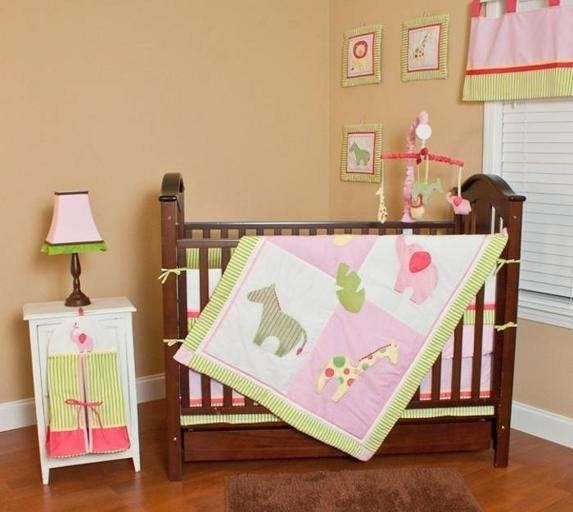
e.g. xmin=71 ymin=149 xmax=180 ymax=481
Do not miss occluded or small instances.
xmin=157 ymin=171 xmax=526 ymax=483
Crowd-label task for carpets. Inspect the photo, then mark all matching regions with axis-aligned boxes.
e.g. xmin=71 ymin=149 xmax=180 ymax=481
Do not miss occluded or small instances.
xmin=223 ymin=465 xmax=485 ymax=512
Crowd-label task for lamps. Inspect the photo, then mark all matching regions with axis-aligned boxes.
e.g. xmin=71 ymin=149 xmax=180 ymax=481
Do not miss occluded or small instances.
xmin=36 ymin=190 xmax=111 ymax=307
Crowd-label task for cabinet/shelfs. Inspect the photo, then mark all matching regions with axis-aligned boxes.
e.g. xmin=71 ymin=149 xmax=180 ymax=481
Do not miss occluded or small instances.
xmin=22 ymin=295 xmax=141 ymax=487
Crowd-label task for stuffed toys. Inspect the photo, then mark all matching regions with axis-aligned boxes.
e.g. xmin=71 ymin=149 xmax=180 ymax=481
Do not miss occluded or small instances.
xmin=409 ymin=194 xmax=425 ymax=221
xmin=446 ymin=191 xmax=472 ymax=216
xmin=375 ymin=187 xmax=388 ymax=224
xmin=411 ymin=177 xmax=443 ymax=206
